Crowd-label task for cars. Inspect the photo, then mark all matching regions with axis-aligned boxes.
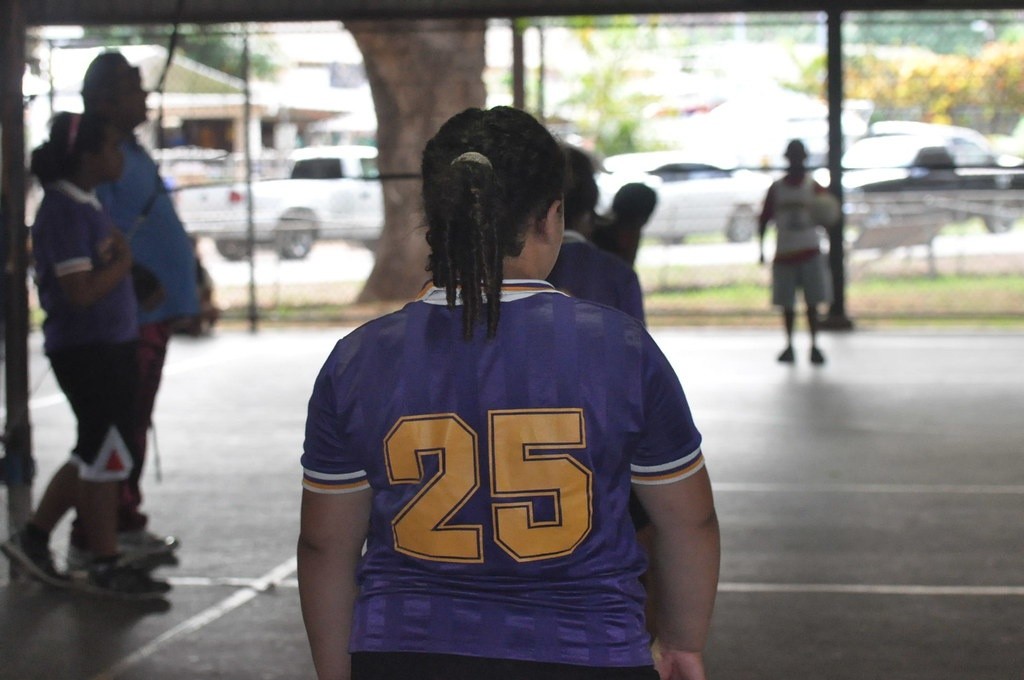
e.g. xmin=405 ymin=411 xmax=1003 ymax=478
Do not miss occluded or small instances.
xmin=592 ymin=149 xmax=773 ymax=246
xmin=839 ymin=119 xmax=1024 ymax=194
xmin=149 ymin=142 xmax=388 ymax=259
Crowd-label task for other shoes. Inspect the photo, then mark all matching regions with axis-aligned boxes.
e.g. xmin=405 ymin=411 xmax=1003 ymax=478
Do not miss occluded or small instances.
xmin=112 ymin=528 xmax=177 ymax=555
xmin=2 ymin=523 xmax=78 ymax=589
xmin=85 ymin=562 xmax=170 ymax=599
xmin=780 ymin=347 xmax=795 ymax=361
xmin=812 ymin=348 xmax=823 ymax=364
xmin=67 ymin=543 xmax=142 ymax=569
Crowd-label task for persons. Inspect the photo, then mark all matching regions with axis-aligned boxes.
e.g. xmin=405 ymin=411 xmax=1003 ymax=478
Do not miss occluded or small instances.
xmin=758 ymin=140 xmax=843 ymax=364
xmin=0 ymin=51 xmax=219 ymax=602
xmin=297 ymin=104 xmax=722 ymax=680
